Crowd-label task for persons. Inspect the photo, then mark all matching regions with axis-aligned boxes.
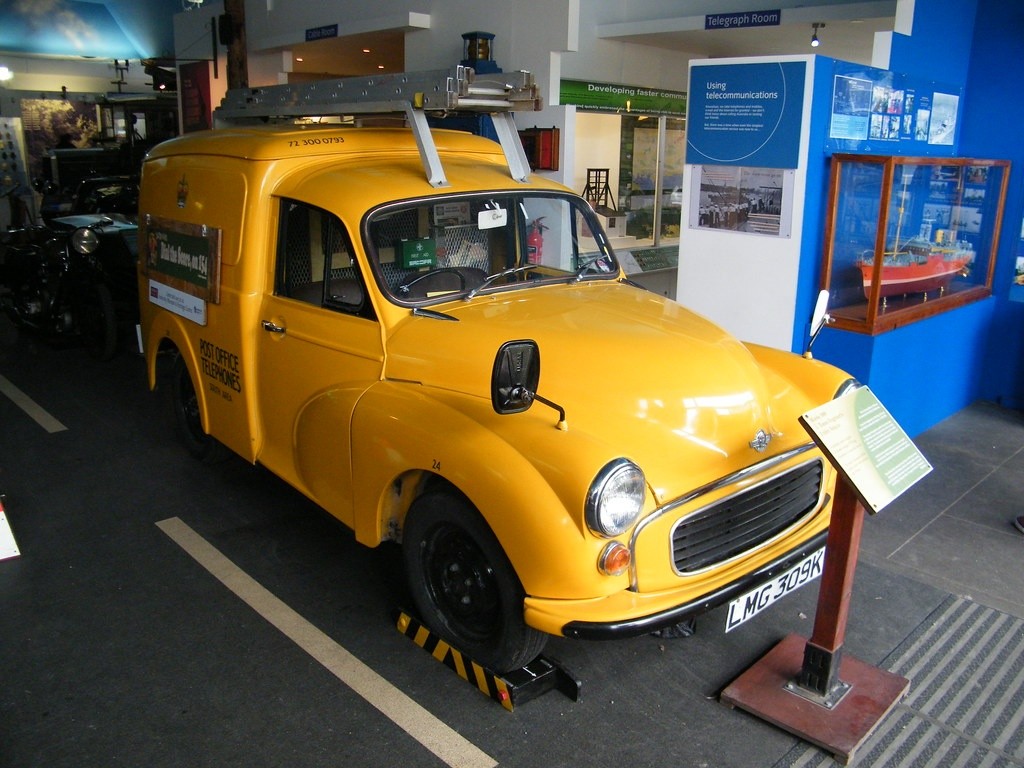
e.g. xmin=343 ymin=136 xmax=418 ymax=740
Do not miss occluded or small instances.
xmin=699 ymin=195 xmax=772 ymax=226
xmin=837 ymin=91 xmax=845 ymax=103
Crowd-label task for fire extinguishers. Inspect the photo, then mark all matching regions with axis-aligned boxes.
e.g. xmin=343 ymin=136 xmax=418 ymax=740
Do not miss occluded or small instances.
xmin=527 ymin=216 xmax=550 ymax=264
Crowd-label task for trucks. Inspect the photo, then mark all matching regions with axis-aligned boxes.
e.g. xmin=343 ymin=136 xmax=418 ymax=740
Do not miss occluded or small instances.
xmin=132 ymin=67 xmax=859 ymax=674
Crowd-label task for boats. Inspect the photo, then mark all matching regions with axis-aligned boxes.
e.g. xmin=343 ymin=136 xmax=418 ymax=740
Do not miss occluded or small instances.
xmin=859 ymin=243 xmax=975 ymax=301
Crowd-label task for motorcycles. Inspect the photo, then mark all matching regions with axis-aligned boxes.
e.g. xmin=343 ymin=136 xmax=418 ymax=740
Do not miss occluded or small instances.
xmin=0 ymin=219 xmax=118 ymax=362
